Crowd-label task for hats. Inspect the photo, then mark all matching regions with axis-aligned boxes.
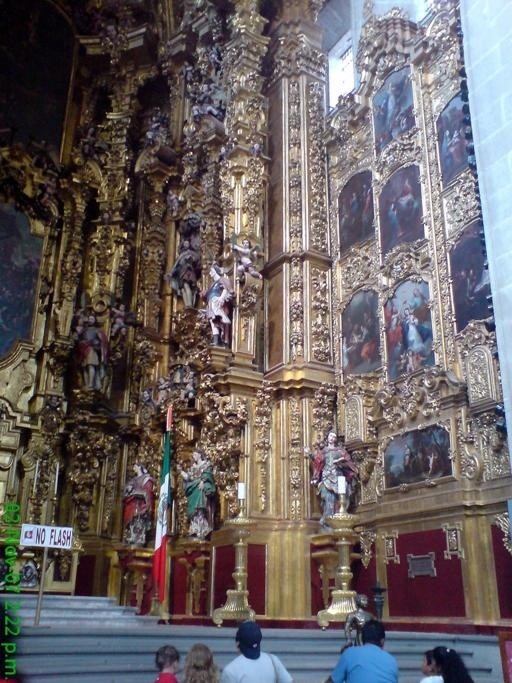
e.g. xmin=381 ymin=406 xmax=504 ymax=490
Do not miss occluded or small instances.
xmin=237 ymin=621 xmax=260 ymax=659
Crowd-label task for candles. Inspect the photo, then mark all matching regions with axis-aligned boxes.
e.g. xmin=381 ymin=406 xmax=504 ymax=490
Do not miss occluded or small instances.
xmin=337 ymin=475 xmax=346 ymax=496
xmin=237 ymin=481 xmax=247 ymax=500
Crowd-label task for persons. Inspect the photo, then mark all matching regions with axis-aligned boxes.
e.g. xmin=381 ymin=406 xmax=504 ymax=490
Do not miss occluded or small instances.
xmin=177 ymin=448 xmax=216 ymax=539
xmin=421 ymin=649 xmax=435 ymax=676
xmin=340 ymin=311 xmax=379 ymax=369
xmin=443 ymin=127 xmax=468 ymax=165
xmin=385 ymin=287 xmax=435 ymax=373
xmin=74 ymin=3 xmax=265 ymax=403
xmin=403 ymin=442 xmax=411 ymax=469
xmin=418 ymin=646 xmax=473 ymax=683
xmin=404 ymin=177 xmax=410 ymax=195
xmin=122 ymin=462 xmax=157 ymax=546
xmin=219 ymin=619 xmax=293 ymax=683
xmin=340 ymin=184 xmax=372 ymax=226
xmin=180 ymin=642 xmax=220 ymax=683
xmin=386 ymin=203 xmax=400 ymax=242
xmin=153 ymin=644 xmax=180 ymax=683
xmin=304 ymin=429 xmax=359 ymax=530
xmin=345 ymin=593 xmax=374 ymax=647
xmin=325 ymin=619 xmax=399 ymax=682
